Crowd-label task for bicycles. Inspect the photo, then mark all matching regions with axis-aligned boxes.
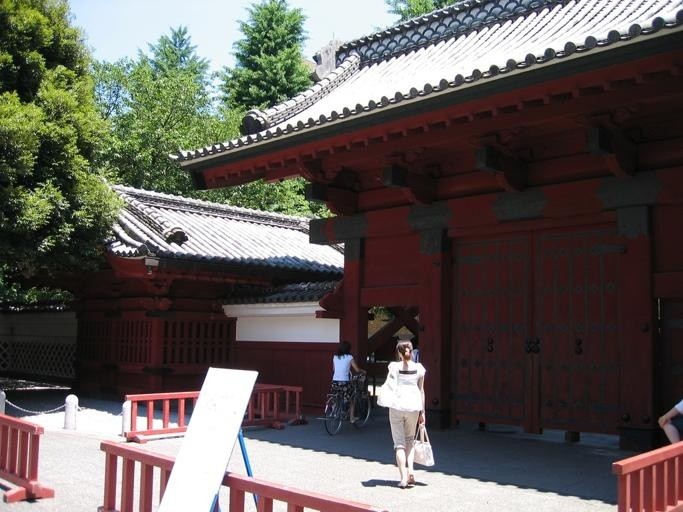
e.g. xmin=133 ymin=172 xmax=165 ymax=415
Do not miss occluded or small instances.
xmin=320 ymin=369 xmax=376 ymax=437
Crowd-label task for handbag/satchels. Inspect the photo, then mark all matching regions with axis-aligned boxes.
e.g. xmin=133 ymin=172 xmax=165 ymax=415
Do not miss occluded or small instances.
xmin=414 ymin=424 xmax=434 ymax=467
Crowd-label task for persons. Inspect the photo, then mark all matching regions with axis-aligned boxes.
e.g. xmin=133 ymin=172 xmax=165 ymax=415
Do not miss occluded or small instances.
xmin=408 ymin=344 xmax=419 ymax=362
xmin=328 ymin=339 xmax=367 ymax=425
xmin=656 ymin=397 xmax=683 ymax=445
xmin=375 ymin=339 xmax=428 ymax=489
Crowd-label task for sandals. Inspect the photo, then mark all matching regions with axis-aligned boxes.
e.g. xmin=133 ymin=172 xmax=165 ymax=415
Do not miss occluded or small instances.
xmin=350 ymin=417 xmax=360 ymax=423
xmin=397 ymin=479 xmax=408 ymax=489
xmin=407 ymin=473 xmax=416 ymax=484
